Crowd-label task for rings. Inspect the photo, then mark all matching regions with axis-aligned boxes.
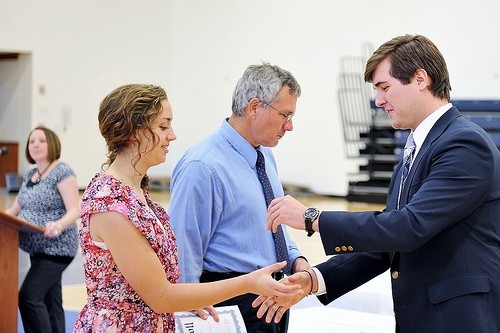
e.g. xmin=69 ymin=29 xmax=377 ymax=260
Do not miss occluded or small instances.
xmin=55 ymin=231 xmax=58 ymax=234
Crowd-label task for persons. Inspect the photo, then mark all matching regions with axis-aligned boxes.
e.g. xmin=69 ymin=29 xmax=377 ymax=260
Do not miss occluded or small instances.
xmin=5 ymin=127 xmax=80 ymax=333
xmin=167 ymin=62 xmax=310 ymax=333
xmin=252 ymin=35 xmax=500 ymax=333
xmin=73 ymin=84 xmax=304 ymax=333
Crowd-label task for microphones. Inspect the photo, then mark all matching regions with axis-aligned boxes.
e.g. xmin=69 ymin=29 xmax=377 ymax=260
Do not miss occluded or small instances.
xmin=1 ymin=146 xmax=9 ymax=155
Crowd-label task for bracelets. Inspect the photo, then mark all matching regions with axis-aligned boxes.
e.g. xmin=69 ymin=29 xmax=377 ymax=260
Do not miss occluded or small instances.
xmin=301 ymin=270 xmax=313 ymax=295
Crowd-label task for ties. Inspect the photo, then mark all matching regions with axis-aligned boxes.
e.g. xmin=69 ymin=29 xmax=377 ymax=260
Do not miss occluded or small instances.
xmin=255 ymin=150 xmax=292 ymax=274
xmin=400 ymin=132 xmax=415 ymax=201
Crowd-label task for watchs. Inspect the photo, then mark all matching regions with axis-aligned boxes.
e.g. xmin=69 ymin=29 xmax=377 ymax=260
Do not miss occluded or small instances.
xmin=303 ymin=207 xmax=319 ymax=237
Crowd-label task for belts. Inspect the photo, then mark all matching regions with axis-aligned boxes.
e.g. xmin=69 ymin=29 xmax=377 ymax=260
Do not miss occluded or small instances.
xmin=199 ymin=270 xmax=284 ymax=281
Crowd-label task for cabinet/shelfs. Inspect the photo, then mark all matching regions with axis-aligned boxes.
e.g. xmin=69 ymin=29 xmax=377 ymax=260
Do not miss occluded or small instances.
xmin=346 ymin=100 xmax=500 ymax=205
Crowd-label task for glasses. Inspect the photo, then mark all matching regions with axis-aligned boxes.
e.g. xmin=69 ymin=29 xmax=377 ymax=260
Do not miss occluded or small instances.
xmin=247 ymin=98 xmax=293 ymax=122
